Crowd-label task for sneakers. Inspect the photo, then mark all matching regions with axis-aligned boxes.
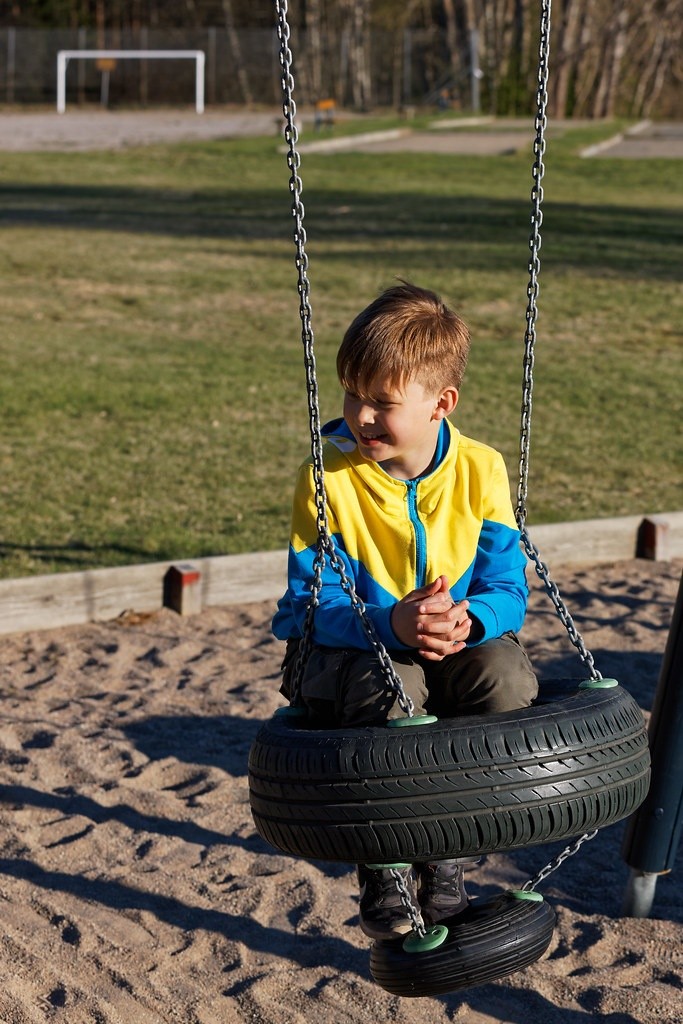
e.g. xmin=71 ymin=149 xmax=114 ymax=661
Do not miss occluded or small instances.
xmin=413 ymin=859 xmax=467 ymax=918
xmin=355 ymin=862 xmax=421 ymax=940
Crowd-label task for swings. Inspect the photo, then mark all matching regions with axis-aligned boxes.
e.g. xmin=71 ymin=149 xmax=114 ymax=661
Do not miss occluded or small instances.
xmin=245 ymin=1 xmax=652 ymax=999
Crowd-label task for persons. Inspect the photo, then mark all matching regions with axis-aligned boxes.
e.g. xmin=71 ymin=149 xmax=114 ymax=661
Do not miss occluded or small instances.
xmin=270 ymin=278 xmax=529 ymax=941
